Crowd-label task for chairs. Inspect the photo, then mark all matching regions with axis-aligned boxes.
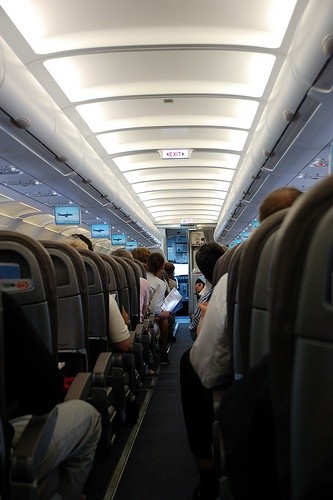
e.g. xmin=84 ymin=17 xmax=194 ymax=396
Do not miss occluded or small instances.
xmin=0 ymin=175 xmax=333 ymax=500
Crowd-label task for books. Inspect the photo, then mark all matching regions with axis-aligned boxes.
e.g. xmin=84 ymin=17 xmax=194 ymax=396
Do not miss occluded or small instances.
xmin=194 ymin=276 xmax=207 ymax=296
xmin=160 ymin=286 xmax=183 ymax=315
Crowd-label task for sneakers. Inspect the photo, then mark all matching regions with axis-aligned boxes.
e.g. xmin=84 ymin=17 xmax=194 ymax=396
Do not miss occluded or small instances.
xmin=160 ymin=352 xmax=170 ymax=366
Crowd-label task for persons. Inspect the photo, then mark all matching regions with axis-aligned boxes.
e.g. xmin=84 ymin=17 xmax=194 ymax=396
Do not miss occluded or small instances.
xmin=1 ymin=397 xmax=105 ymax=500
xmin=175 ymin=185 xmax=333 ymax=499
xmin=53 ymin=227 xmax=181 ymax=375
xmin=142 ymin=252 xmax=172 ymax=367
xmin=59 ymin=237 xmax=135 ymax=352
xmin=189 ymin=240 xmax=230 ymax=349
